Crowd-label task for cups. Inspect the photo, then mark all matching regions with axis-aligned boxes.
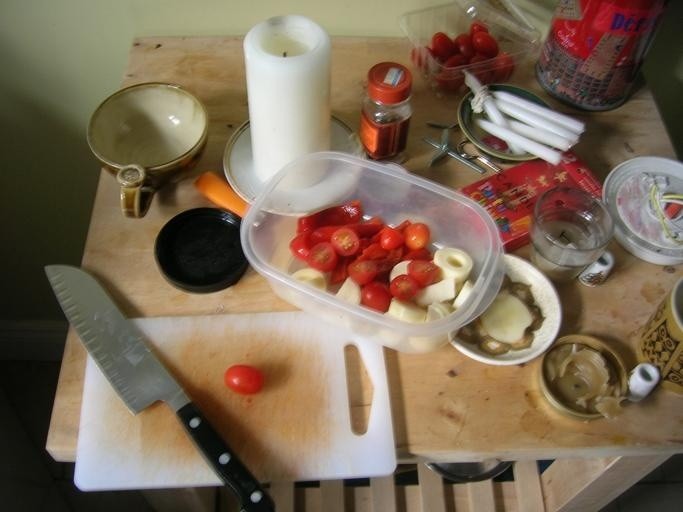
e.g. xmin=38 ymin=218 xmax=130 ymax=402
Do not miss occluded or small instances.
xmin=526 ymin=188 xmax=613 ymax=284
xmin=635 ymin=279 xmax=683 ymax=398
xmin=86 ymin=82 xmax=211 ymax=216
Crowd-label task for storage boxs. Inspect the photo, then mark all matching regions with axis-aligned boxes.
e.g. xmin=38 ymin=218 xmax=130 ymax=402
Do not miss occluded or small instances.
xmin=238 ymin=146 xmax=506 ymax=359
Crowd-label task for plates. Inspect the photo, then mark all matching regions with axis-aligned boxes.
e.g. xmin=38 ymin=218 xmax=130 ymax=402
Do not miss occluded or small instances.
xmin=602 ymin=151 xmax=683 ymax=267
xmin=422 ymin=461 xmax=516 ymax=485
xmin=457 ymin=84 xmax=555 ymax=161
xmin=449 ymin=252 xmax=564 ymax=368
xmin=219 ymin=112 xmax=367 ymax=219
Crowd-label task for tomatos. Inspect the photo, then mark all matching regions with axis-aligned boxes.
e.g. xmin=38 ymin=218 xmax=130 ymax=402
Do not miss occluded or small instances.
xmin=289 ymin=201 xmax=440 ymax=312
xmin=411 ymin=21 xmax=515 ymax=94
xmin=224 ymin=365 xmax=265 ymax=394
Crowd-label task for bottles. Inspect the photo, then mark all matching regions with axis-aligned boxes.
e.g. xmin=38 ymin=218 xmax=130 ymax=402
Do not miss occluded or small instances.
xmin=533 ymin=0 xmax=665 ymax=113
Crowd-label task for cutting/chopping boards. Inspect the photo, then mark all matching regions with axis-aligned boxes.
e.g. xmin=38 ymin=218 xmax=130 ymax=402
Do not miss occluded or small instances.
xmin=71 ymin=316 xmax=399 ymax=491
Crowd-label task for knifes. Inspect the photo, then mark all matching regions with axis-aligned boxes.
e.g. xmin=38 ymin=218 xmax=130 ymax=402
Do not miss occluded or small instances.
xmin=44 ymin=262 xmax=279 ymax=512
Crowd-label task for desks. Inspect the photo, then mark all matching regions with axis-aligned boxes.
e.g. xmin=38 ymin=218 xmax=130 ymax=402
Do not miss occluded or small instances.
xmin=40 ymin=31 xmax=680 ymax=512
